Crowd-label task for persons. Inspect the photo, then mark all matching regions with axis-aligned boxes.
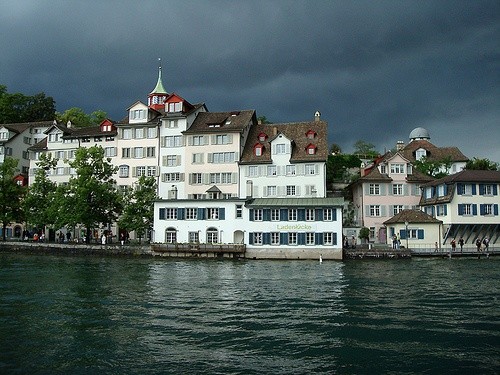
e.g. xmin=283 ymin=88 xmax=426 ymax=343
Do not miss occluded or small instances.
xmin=24 ymin=231 xmax=125 ymax=245
xmin=475 ymin=236 xmax=490 ymax=252
xmin=452 ymin=238 xmax=456 ymax=251
xmin=435 ymin=242 xmax=439 ymax=253
xmin=458 ymin=236 xmax=464 ymax=252
xmin=392 ymin=233 xmax=401 ymax=249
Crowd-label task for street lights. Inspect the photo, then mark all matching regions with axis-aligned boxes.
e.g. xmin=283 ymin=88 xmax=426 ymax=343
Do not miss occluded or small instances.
xmin=175 ymin=230 xmax=179 ymax=243
xmin=404 ymin=219 xmax=409 ymax=249
xmin=206 ymin=231 xmax=209 ymax=244
xmin=242 ymin=230 xmax=246 ymax=245
xmin=198 ymin=230 xmax=202 ymax=244
xmin=152 ymin=230 xmax=155 ymax=244
xmin=220 ymin=230 xmax=224 ymax=244
